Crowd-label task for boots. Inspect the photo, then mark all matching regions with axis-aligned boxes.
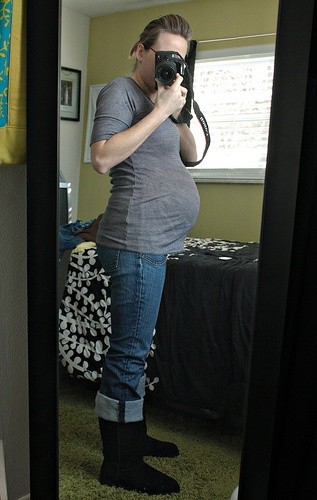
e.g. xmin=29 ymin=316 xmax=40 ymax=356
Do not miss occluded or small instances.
xmin=98 ymin=417 xmax=180 ymax=494
xmin=138 ymin=418 xmax=179 ymax=457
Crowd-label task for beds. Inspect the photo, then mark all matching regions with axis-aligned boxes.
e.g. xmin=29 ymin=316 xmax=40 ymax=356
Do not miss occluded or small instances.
xmin=57 ymin=235 xmax=260 ymax=428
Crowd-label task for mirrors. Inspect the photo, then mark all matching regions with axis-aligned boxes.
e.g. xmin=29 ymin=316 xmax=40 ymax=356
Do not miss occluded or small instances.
xmin=22 ymin=1 xmax=315 ymax=500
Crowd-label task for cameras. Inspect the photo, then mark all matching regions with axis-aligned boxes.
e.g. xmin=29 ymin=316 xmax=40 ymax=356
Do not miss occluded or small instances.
xmin=155 ymin=51 xmax=184 ymax=90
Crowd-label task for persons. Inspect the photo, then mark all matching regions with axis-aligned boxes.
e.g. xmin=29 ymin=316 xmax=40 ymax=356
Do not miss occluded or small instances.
xmin=88 ymin=14 xmax=200 ymax=492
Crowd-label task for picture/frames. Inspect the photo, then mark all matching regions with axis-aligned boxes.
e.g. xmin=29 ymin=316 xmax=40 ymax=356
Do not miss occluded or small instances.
xmin=82 ymin=83 xmax=109 ymax=164
xmin=60 ymin=66 xmax=81 ymax=121
